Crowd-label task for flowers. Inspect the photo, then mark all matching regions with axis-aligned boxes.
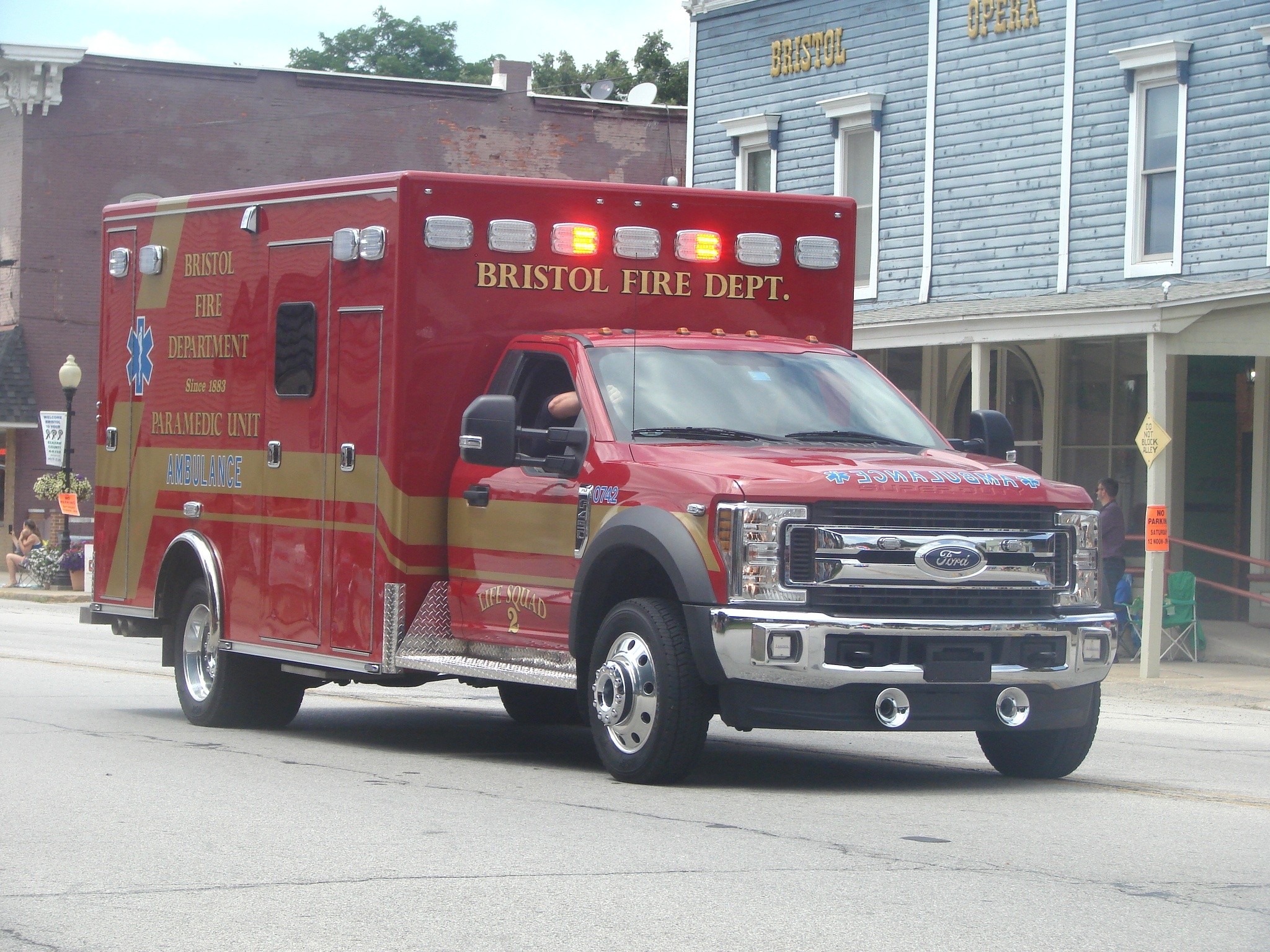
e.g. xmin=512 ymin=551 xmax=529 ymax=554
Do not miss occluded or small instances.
xmin=60 ymin=541 xmax=94 ymax=573
xmin=33 ymin=470 xmax=93 ymax=502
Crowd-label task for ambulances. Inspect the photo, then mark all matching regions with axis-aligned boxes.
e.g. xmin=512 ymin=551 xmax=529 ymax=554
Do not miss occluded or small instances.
xmin=80 ymin=170 xmax=1119 ymax=780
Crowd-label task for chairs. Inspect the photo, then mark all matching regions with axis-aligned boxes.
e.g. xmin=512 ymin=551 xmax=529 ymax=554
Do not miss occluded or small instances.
xmin=1095 ymin=573 xmax=1133 ymax=657
xmin=1121 ymin=570 xmax=1205 ymax=662
xmin=17 ymin=564 xmax=44 ymax=588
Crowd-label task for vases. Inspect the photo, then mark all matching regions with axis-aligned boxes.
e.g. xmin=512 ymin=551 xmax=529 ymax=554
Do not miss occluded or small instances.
xmin=69 ymin=570 xmax=85 ymax=591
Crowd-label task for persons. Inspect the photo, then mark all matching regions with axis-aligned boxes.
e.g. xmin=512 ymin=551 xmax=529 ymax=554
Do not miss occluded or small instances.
xmin=16 ymin=529 xmax=34 ymax=557
xmin=3 ymin=519 xmax=43 ymax=588
xmin=530 ymin=351 xmax=646 ymax=479
xmin=1095 ymin=477 xmax=1141 ymax=664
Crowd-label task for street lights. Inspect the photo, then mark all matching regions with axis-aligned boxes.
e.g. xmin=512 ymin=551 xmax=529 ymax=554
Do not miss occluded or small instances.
xmin=54 ymin=354 xmax=82 ymax=585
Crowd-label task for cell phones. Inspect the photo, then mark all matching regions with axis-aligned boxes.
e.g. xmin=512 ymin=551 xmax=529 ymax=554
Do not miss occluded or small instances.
xmin=8 ymin=525 xmax=13 ymax=534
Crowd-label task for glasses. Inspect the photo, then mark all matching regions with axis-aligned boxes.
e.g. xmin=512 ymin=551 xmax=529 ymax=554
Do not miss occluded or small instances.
xmin=1096 ymin=488 xmax=1103 ymax=491
